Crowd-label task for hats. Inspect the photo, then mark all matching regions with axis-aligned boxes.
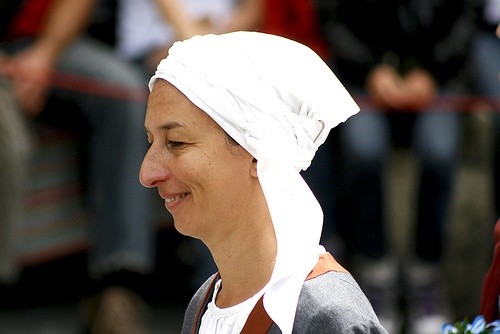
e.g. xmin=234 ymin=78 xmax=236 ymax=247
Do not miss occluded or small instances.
xmin=149 ymin=30 xmax=360 ymax=334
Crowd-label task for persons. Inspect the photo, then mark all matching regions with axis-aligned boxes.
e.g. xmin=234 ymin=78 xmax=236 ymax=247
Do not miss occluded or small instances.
xmin=139 ymin=33 xmax=392 ymax=334
xmin=113 ymin=0 xmax=332 ymax=270
xmin=314 ymin=1 xmax=499 ymax=334
xmin=1 ymin=0 xmax=155 ymax=334
